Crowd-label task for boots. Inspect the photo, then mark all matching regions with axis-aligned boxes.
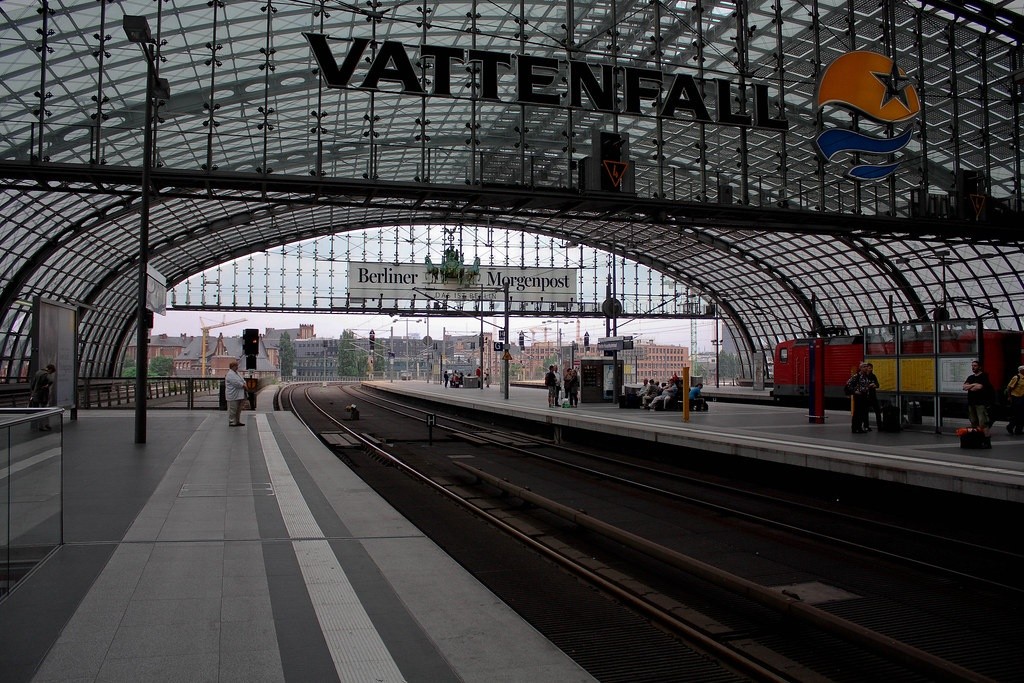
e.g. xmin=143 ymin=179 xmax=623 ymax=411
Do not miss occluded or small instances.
xmin=852 ymin=417 xmax=872 ymax=434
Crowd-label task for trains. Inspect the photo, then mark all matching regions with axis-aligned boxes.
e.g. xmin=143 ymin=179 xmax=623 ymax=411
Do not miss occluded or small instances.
xmin=769 ymin=329 xmax=1024 ymax=420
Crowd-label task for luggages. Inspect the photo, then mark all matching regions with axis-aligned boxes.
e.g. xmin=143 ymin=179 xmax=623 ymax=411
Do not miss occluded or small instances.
xmin=882 ymin=403 xmax=900 ymax=433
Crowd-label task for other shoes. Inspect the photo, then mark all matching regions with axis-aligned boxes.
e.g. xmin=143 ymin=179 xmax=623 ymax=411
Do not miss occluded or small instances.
xmin=644 ymin=406 xmax=651 ymax=409
xmin=571 ymin=405 xmax=575 ymax=407
xmin=574 ymin=405 xmax=577 ymax=408
xmin=1015 ymin=430 xmax=1024 ymax=435
xmin=39 ymin=425 xmax=51 ymax=431
xmin=31 ymin=427 xmax=38 ymax=431
xmin=229 ymin=422 xmax=245 ymax=426
xmin=555 ymin=404 xmax=561 ymax=407
xmin=1007 ymin=425 xmax=1014 ymax=436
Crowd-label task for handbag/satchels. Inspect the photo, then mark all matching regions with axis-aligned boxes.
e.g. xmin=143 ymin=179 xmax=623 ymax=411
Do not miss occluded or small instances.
xmin=562 ymin=403 xmax=570 ymax=408
xmin=1004 ymin=375 xmax=1020 ymax=396
xmin=29 ymin=397 xmax=34 ymax=406
xmin=959 ymin=425 xmax=992 ymax=449
xmin=844 ymin=372 xmax=860 ymax=395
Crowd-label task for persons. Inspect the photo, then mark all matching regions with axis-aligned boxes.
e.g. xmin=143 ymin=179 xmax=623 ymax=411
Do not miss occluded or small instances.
xmin=444 ymin=371 xmax=449 ymax=387
xmin=224 ymin=362 xmax=249 ymax=427
xmin=1006 ymin=366 xmax=1024 ymax=436
xmin=963 ymin=360 xmax=989 ymax=428
xmin=484 ymin=374 xmax=490 ymax=387
xmin=476 ymin=366 xmax=481 ymax=388
xmin=637 ymin=378 xmax=708 ymax=412
xmin=848 ymin=363 xmax=879 ymax=433
xmin=545 ymin=365 xmax=581 ymax=408
xmin=450 ymin=370 xmax=464 ymax=387
xmin=30 ymin=364 xmax=56 ymax=432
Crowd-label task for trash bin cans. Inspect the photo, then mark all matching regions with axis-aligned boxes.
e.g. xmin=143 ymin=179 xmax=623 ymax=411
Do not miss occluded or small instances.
xmin=220 ymin=381 xmax=227 ymax=411
xmin=908 ymin=401 xmax=922 ymax=425
xmin=875 ymin=397 xmax=900 ymax=433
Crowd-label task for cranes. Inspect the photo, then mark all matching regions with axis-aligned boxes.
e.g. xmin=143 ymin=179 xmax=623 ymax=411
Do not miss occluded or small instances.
xmin=199 ymin=316 xmax=248 ymax=375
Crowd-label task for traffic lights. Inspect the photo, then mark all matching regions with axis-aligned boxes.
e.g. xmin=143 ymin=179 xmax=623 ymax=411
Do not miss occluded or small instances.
xmin=247 ymin=329 xmax=260 ymax=355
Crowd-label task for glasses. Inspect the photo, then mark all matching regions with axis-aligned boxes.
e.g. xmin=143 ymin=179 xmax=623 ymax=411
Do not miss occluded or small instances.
xmin=862 ymin=365 xmax=868 ymax=368
